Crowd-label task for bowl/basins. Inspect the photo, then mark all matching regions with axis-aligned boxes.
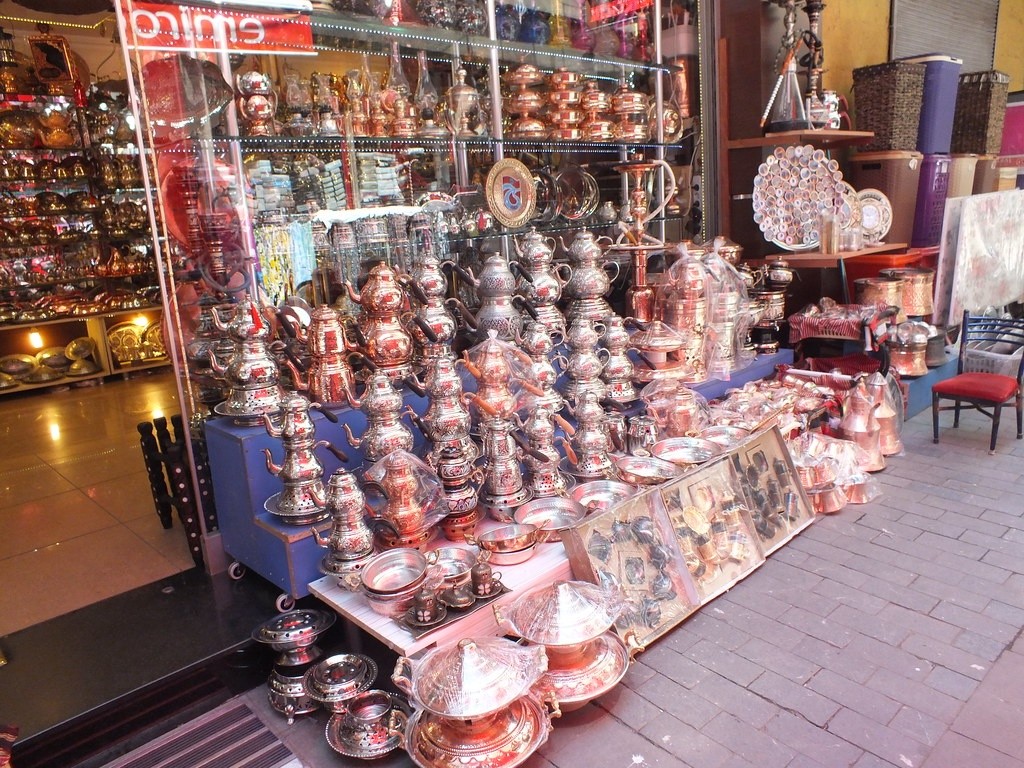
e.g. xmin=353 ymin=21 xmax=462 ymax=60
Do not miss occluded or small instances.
xmin=570 ymin=481 xmax=636 ymax=516
xmin=651 ymin=437 xmax=722 ymax=465
xmin=702 ymin=426 xmax=749 ymax=451
xmin=615 ymin=456 xmax=682 ymax=487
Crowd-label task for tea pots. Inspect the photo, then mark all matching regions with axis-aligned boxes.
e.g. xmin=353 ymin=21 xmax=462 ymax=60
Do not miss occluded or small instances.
xmin=208 ymin=223 xmax=658 ymax=579
xmin=37 ymin=109 xmax=76 ymax=147
xmin=668 ymin=260 xmax=706 ymax=300
xmin=731 ymin=256 xmax=803 ymax=289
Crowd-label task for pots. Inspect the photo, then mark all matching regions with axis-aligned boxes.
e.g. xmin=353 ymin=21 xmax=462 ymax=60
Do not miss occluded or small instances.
xmin=502 ymin=497 xmax=597 ymax=543
xmin=880 ymin=268 xmax=936 ymax=316
xmin=855 ymin=277 xmax=908 ymax=324
xmin=346 ymin=549 xmax=440 ymax=615
xmin=464 ymin=519 xmax=552 ymax=566
xmin=425 ymin=548 xmax=493 ymax=581
xmin=747 ymin=289 xmax=787 ymax=320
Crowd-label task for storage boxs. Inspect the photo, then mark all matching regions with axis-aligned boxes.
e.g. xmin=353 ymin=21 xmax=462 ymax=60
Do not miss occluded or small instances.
xmin=992 ymin=167 xmax=1019 ymax=191
xmin=945 ymin=153 xmax=979 ymax=199
xmin=913 ymin=154 xmax=953 ymax=247
xmin=842 ymin=250 xmax=922 ymax=304
xmin=894 ymin=53 xmax=964 ymax=153
xmin=972 ymin=154 xmax=1002 ymax=196
xmin=910 ymin=246 xmax=940 ymax=323
xmin=848 ymin=150 xmax=926 ymax=250
xmin=964 ymin=348 xmax=1022 ymax=378
xmin=648 ymin=23 xmax=700 ymax=118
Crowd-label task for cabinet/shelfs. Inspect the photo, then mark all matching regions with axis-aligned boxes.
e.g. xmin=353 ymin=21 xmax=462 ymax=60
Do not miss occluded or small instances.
xmin=178 ymin=0 xmax=684 ymax=312
xmin=0 ymin=78 xmax=172 ymax=394
xmin=720 ymin=38 xmax=909 ymax=269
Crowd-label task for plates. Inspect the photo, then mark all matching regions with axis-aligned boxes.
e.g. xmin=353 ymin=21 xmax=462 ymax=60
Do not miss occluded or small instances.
xmin=264 ymin=492 xmax=328 ymax=525
xmin=214 ymin=402 xmax=280 ymax=425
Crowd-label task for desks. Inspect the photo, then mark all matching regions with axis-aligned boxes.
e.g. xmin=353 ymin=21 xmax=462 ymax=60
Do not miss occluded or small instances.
xmin=789 ymin=304 xmax=900 ymax=375
xmin=307 ymin=474 xmax=633 ymax=658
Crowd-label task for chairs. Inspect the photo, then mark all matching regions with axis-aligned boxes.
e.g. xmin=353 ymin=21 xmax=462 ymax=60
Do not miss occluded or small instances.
xmin=931 ymin=309 xmax=1024 ymax=456
